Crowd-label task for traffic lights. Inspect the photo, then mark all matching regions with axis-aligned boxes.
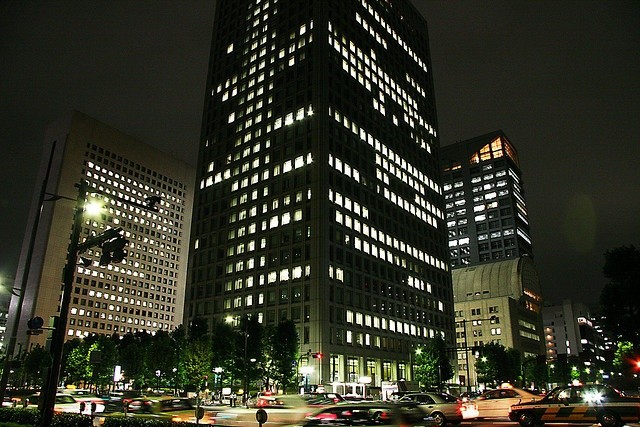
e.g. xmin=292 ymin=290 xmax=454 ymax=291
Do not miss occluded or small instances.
xmin=312 ymin=353 xmax=323 ymax=359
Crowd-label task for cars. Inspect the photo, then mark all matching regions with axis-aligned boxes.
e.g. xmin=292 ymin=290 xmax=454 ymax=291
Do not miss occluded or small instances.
xmin=35 ymin=388 xmax=104 ymax=406
xmin=398 ymin=393 xmax=479 ymax=427
xmin=302 ymin=392 xmax=345 ymax=405
xmin=299 ymin=405 xmax=410 ymax=427
xmin=470 ymin=388 xmax=544 ymax=421
xmin=8 ymin=392 xmax=91 ymax=415
xmin=245 ymin=392 xmax=285 ymax=409
xmin=507 ymin=385 xmax=640 ymax=427
xmin=111 ymin=394 xmax=210 ymax=425
xmin=105 ymin=389 xmax=153 ymax=414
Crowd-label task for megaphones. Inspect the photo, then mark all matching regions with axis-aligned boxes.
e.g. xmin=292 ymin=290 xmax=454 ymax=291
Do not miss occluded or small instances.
xmin=80 ymin=256 xmax=92 ymax=268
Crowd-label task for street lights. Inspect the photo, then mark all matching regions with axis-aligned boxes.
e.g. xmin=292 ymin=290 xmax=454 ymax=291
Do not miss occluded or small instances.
xmin=1 ymin=139 xmax=102 ymax=410
